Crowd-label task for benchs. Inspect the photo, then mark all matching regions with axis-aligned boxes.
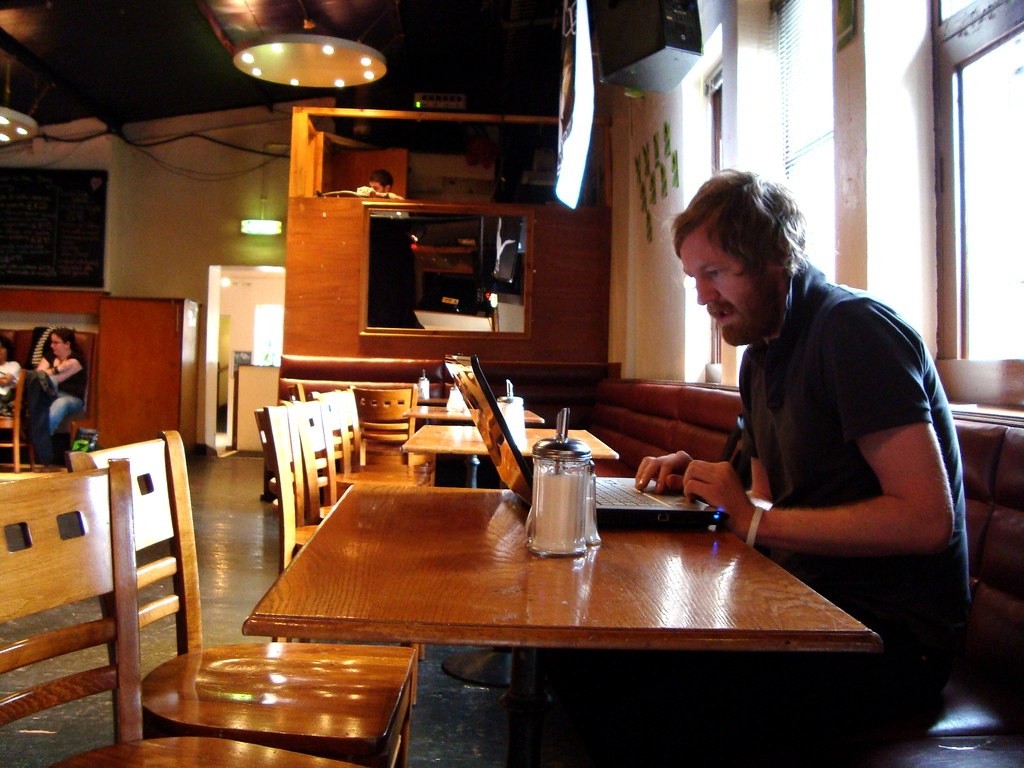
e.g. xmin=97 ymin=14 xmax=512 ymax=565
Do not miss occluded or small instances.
xmin=588 ymin=378 xmax=1024 ymax=768
xmin=0 ymin=328 xmax=98 ymax=449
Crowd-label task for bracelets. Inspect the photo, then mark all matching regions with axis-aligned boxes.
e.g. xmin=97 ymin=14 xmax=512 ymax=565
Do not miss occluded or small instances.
xmin=51 ymin=366 xmax=59 ymax=375
xmin=746 ymin=506 xmax=764 ymax=547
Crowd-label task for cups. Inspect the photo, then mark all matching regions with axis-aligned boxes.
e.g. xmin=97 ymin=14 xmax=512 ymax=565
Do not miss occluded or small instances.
xmin=446 ymin=383 xmax=464 ymax=412
xmin=497 ymin=379 xmax=526 ymax=451
xmin=523 ymin=407 xmax=592 ymax=557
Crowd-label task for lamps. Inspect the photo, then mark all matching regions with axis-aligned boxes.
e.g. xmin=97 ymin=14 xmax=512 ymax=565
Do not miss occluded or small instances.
xmin=240 ymin=163 xmax=281 ymax=235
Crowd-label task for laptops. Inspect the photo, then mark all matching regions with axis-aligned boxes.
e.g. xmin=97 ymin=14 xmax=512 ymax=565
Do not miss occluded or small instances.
xmin=441 ymin=353 xmax=729 ymax=534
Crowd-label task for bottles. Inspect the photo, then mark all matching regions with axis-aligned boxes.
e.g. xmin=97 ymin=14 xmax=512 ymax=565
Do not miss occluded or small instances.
xmin=419 ymin=370 xmax=430 ymax=400
xmin=583 ymin=460 xmax=602 ymax=546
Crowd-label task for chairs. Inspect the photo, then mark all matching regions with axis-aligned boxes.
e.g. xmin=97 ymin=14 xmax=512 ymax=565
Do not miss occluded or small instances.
xmin=253 ymin=382 xmax=438 ymax=584
xmin=70 ymin=428 xmax=416 ymax=768
xmin=0 ymin=457 xmax=361 ymax=768
xmin=1 ymin=368 xmax=37 ymax=475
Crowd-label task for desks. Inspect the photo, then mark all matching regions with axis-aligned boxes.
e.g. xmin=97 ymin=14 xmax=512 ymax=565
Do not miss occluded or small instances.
xmin=399 ymin=423 xmax=622 ymax=491
xmin=404 ymin=407 xmax=547 ymax=427
xmin=241 ymin=480 xmax=881 ymax=767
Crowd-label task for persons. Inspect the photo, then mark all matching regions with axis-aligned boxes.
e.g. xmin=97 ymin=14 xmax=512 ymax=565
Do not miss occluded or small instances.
xmin=566 ymin=170 xmax=970 ymax=768
xmin=0 ymin=334 xmax=21 ymax=416
xmin=35 ymin=328 xmax=88 ymax=435
xmin=356 ymin=168 xmax=405 ymax=200
